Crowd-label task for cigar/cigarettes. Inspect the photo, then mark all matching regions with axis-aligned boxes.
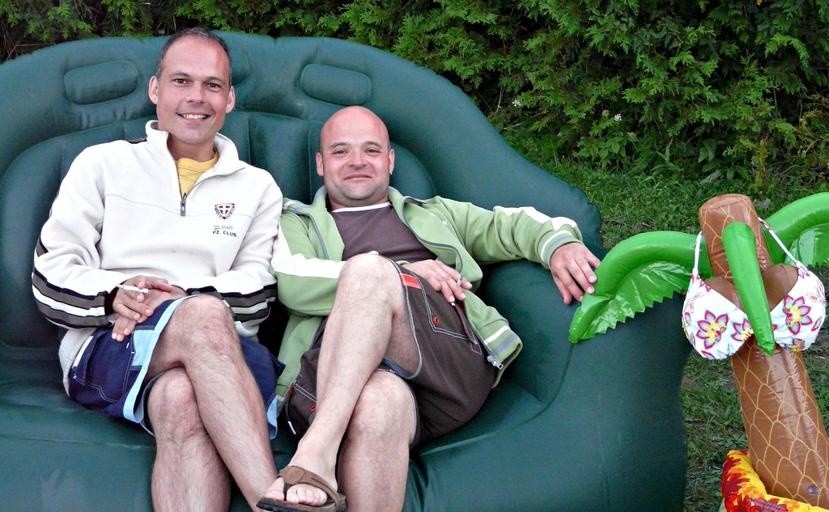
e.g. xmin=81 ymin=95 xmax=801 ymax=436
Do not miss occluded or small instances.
xmin=115 ymin=282 xmax=148 ymax=294
xmin=457 ymin=276 xmax=466 ymax=288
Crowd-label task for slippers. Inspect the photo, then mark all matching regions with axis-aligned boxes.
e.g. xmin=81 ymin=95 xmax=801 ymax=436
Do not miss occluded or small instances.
xmin=258 ymin=465 xmax=347 ymax=512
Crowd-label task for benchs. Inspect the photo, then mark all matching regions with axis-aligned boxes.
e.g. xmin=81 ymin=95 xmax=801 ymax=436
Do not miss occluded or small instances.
xmin=0 ymin=32 xmax=696 ymax=512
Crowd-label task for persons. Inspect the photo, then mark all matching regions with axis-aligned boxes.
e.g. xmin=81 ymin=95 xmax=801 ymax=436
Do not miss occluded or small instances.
xmin=256 ymin=105 xmax=602 ymax=512
xmin=32 ymin=28 xmax=282 ymax=511
